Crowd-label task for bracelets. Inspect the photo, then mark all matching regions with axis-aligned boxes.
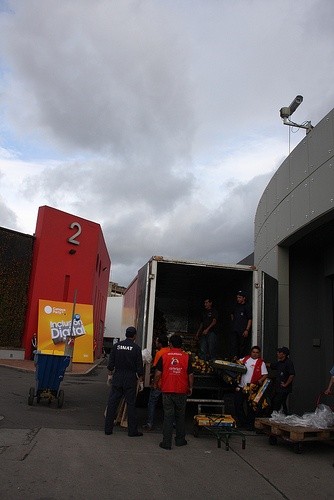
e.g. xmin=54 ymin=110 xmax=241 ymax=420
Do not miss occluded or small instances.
xmin=245 ymin=329 xmax=249 ymax=332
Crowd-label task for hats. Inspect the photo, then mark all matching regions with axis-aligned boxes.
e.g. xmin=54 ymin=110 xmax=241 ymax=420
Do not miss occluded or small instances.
xmin=277 ymin=347 xmax=290 ymax=355
xmin=126 ymin=328 xmax=136 ymax=337
xmin=236 ymin=289 xmax=248 ymax=296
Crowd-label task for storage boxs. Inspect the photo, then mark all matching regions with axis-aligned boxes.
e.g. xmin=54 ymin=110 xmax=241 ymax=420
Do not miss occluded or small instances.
xmin=253 ymin=378 xmax=271 ymax=404
xmin=195 ymin=414 xmax=236 ymax=427
xmin=214 ymin=360 xmax=247 ymax=374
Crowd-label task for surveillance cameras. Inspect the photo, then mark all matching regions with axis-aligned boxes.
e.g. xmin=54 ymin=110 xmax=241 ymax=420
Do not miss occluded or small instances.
xmin=289 ymin=95 xmax=303 ymax=115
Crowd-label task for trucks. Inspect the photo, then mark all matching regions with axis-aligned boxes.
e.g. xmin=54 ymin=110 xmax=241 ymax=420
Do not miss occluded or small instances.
xmin=119 ymin=256 xmax=265 ymax=409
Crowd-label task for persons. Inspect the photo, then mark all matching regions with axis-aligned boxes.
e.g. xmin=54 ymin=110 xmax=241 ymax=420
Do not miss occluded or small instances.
xmin=196 ymin=297 xmax=219 ymax=359
xmin=152 ymin=334 xmax=193 ymax=449
xmin=324 ymin=366 xmax=334 ymax=395
xmin=265 ymin=347 xmax=295 ymax=416
xmin=229 ymin=290 xmax=252 ymax=357
xmin=235 ymin=346 xmax=269 ymax=420
xmin=105 ymin=327 xmax=143 ymax=437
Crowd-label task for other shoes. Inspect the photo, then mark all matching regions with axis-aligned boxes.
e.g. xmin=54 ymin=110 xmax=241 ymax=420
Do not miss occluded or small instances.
xmin=177 ymin=440 xmax=188 ymax=447
xmin=104 ymin=430 xmax=113 ymax=434
xmin=160 ymin=442 xmax=172 ymax=449
xmin=142 ymin=422 xmax=154 ymax=429
xmin=128 ymin=432 xmax=143 ymax=437
